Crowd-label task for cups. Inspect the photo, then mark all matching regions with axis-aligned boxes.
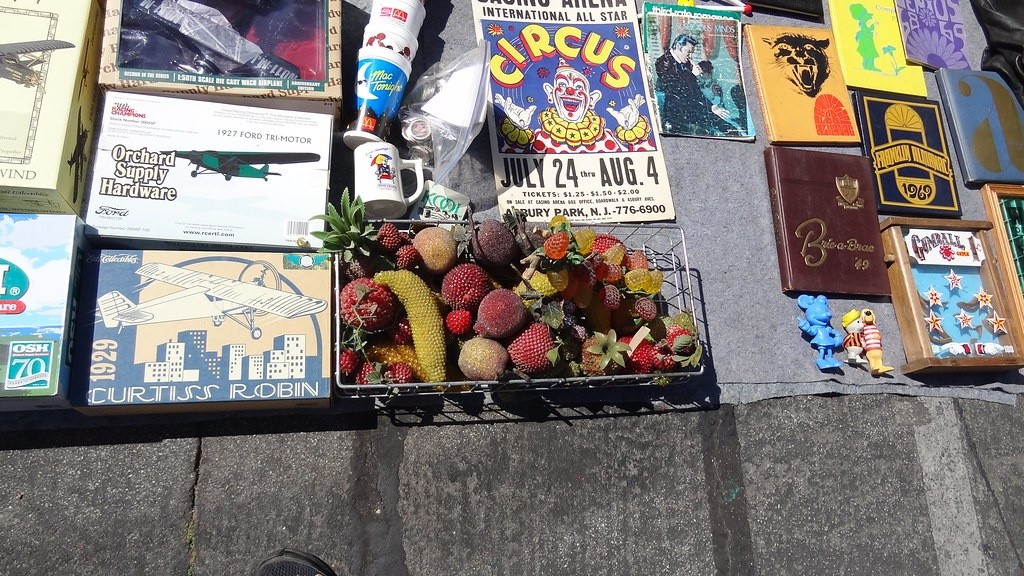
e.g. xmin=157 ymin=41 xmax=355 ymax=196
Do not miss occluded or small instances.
xmin=351 ymin=140 xmax=424 ymax=220
xmin=343 ymin=0 xmax=429 ymax=150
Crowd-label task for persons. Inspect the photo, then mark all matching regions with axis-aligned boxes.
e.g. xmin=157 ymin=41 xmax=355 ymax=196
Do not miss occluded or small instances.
xmin=655 ymin=34 xmax=740 ymax=137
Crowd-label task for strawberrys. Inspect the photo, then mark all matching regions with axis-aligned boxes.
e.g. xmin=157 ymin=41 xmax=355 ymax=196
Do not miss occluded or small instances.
xmin=535 ymin=215 xmax=663 ymax=320
xmin=651 ymin=329 xmax=694 ymax=371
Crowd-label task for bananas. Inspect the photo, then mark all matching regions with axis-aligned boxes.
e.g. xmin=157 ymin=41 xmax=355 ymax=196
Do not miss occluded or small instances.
xmin=373 ymin=270 xmax=447 ymax=381
